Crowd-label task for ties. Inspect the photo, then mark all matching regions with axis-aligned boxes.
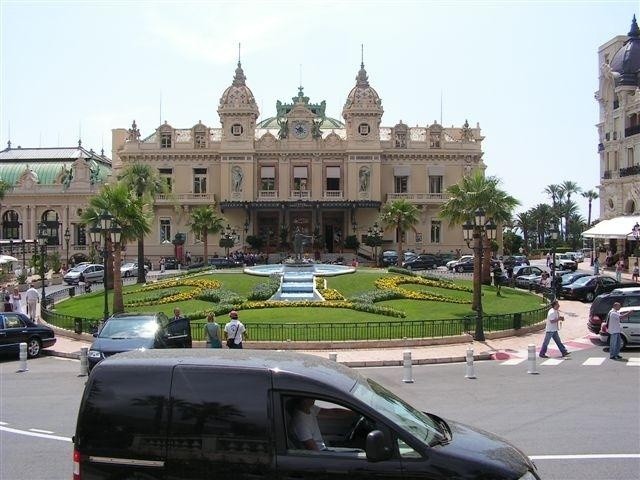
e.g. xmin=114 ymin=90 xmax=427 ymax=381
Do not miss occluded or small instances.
xmin=176 ymin=317 xmax=178 ymax=319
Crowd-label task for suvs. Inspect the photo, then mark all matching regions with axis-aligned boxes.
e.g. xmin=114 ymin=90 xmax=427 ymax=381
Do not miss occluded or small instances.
xmin=87 ymin=307 xmax=194 ymax=377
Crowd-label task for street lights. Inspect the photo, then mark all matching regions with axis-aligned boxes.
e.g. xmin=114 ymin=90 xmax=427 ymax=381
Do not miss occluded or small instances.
xmin=221 ymin=224 xmax=236 ymax=266
xmin=228 ymin=230 xmax=240 ymax=259
xmin=36 ymin=221 xmax=49 ymax=304
xmin=90 ymin=213 xmax=121 ymax=320
xmin=352 ymin=219 xmax=358 ymax=254
xmin=265 ymin=226 xmax=274 ymax=255
xmin=367 ymin=221 xmax=385 ymax=267
xmin=462 ymin=203 xmax=497 ymax=341
xmin=336 ymin=227 xmax=343 ymax=254
xmin=549 ymin=220 xmax=559 ymax=293
xmin=632 ymin=221 xmax=640 ymax=271
xmin=64 ymin=225 xmax=69 ymax=270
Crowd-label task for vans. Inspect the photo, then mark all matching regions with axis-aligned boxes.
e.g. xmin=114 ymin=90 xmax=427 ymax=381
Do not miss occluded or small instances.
xmin=63 ymin=264 xmax=106 ymax=284
xmin=187 ymin=258 xmax=234 ymax=269
xmin=71 ymin=346 xmax=543 ymax=480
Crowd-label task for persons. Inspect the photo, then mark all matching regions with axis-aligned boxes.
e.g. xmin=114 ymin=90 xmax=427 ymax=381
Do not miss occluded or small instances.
xmin=223 ymin=310 xmax=249 ymax=349
xmin=167 ymin=307 xmax=187 ymax=336
xmin=407 ymin=246 xmax=553 ymax=292
xmin=78 ymin=272 xmax=85 ymax=294
xmin=590 ymin=247 xmax=639 ymax=284
xmin=607 ymin=302 xmax=634 ymax=359
xmin=539 ymin=301 xmax=571 ymax=358
xmin=202 ymin=312 xmax=222 ymax=349
xmin=61 ymin=256 xmax=74 ymax=278
xmin=144 ymin=249 xmax=361 ymax=271
xmin=0 ymin=282 xmax=39 ymax=322
xmin=292 ymin=397 xmax=335 ymax=451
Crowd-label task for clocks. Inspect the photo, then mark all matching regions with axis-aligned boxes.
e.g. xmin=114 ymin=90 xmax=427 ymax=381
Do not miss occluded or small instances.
xmin=292 ymin=121 xmax=310 ymax=140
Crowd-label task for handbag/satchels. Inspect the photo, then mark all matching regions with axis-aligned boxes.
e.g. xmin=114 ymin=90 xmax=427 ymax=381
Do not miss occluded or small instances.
xmin=605 ymin=311 xmax=614 ymax=329
xmin=227 ymin=324 xmax=241 ymax=349
xmin=205 ymin=324 xmax=223 ymax=348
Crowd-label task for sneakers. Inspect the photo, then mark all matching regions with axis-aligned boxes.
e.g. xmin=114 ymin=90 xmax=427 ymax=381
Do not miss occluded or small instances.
xmin=609 ymin=354 xmax=623 ymax=360
xmin=561 ymin=351 xmax=572 ymax=357
xmin=539 ymin=353 xmax=548 ymax=358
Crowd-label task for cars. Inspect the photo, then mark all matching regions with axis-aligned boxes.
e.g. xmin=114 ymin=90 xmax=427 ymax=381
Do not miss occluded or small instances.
xmin=0 ymin=313 xmax=58 ymax=357
xmin=113 ymin=262 xmax=149 ymax=278
xmin=380 ymin=249 xmax=640 ymax=350
xmin=67 ymin=261 xmax=94 ymax=272
xmin=159 ymin=256 xmax=181 ymax=271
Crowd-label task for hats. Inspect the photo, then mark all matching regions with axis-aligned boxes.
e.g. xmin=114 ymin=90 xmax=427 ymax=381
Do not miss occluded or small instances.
xmin=229 ymin=311 xmax=238 ymax=318
